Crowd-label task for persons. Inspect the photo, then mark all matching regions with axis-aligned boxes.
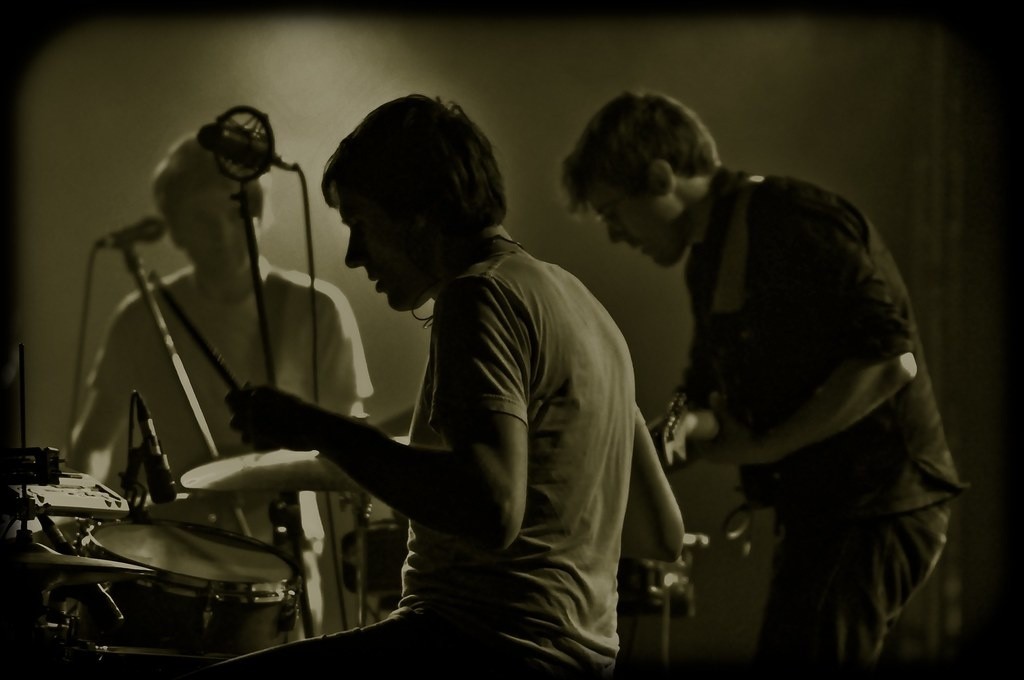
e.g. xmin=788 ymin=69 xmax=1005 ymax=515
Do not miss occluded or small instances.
xmin=64 ymin=92 xmax=964 ymax=680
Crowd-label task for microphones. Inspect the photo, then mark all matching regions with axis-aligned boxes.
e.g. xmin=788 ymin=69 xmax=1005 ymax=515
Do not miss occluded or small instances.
xmin=136 ymin=396 xmax=177 ymax=504
xmin=36 ymin=515 xmax=123 ymax=624
xmin=197 ymin=123 xmax=299 ymax=172
xmin=95 ymin=215 xmax=166 ymax=247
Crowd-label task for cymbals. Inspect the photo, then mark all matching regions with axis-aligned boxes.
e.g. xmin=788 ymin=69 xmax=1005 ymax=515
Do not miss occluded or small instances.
xmin=0 ymin=536 xmax=159 ymax=591
xmin=179 ymin=433 xmax=412 ymax=495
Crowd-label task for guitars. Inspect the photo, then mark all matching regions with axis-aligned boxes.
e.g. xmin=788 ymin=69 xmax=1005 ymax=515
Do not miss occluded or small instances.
xmin=659 ymin=391 xmax=723 ymax=468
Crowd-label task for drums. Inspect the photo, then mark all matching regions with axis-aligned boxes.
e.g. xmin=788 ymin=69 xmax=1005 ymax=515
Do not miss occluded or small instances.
xmin=77 ymin=518 xmax=304 ymax=656
